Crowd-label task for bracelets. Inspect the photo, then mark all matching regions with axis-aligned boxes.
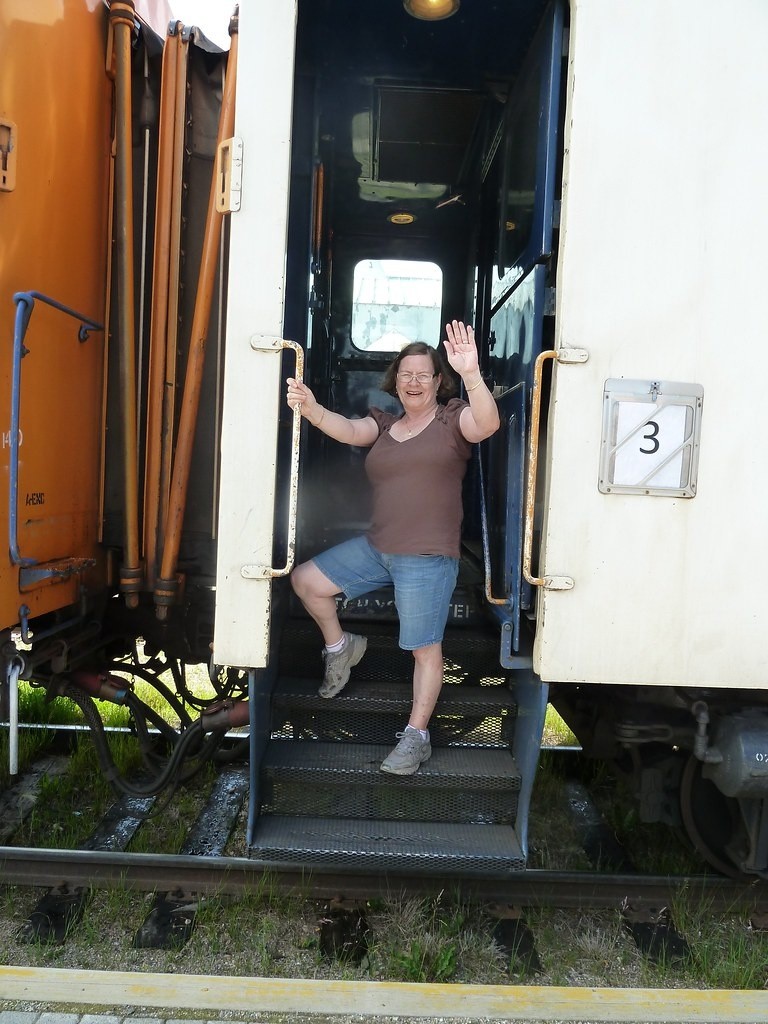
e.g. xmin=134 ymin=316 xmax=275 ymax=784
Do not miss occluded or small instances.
xmin=466 ymin=377 xmax=483 ymax=391
xmin=312 ymin=407 xmax=325 ymax=427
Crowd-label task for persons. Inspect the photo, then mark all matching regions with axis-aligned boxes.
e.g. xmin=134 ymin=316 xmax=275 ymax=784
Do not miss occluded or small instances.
xmin=287 ymin=320 xmax=501 ymax=774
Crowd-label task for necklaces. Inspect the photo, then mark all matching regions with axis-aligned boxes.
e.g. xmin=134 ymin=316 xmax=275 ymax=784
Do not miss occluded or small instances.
xmin=406 ymin=418 xmax=413 ymax=436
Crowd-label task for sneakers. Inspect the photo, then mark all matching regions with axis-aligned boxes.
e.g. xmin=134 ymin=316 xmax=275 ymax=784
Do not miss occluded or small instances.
xmin=317 ymin=630 xmax=368 ymax=700
xmin=379 ymin=723 xmax=433 ymax=777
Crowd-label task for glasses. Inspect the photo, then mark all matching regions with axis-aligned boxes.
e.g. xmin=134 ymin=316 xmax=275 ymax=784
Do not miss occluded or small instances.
xmin=396 ymin=372 xmax=440 ymax=383
